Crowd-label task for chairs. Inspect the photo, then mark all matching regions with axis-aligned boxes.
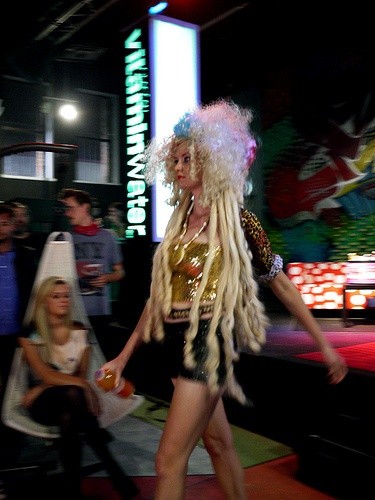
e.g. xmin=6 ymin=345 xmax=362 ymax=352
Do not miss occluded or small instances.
xmin=2 ymin=231 xmax=146 ymax=478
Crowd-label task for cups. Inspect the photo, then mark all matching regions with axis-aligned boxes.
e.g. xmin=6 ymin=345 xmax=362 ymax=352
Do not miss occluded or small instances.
xmin=94 ymin=369 xmax=136 ymax=399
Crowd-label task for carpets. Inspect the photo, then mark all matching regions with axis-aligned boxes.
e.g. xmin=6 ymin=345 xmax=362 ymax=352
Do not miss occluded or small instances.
xmin=37 ymin=392 xmax=299 ymax=478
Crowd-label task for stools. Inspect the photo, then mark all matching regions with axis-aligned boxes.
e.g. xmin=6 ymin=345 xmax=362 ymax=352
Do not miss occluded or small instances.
xmin=341 ymin=283 xmax=375 ymax=327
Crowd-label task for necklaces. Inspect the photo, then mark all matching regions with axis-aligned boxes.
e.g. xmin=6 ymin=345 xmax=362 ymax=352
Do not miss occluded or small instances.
xmin=171 ymin=201 xmax=209 ymax=266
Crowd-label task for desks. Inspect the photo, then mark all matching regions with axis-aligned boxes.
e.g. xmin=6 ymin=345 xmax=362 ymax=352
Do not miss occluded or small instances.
xmin=287 ymin=261 xmax=375 ymax=310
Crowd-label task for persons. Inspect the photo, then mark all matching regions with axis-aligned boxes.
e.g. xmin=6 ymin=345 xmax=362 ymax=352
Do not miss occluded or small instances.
xmin=15 ymin=276 xmax=139 ymax=500
xmin=101 ymin=102 xmax=347 ymax=500
xmin=58 ymin=189 xmax=124 ymax=337
xmin=0 ymin=201 xmax=39 ymax=383
